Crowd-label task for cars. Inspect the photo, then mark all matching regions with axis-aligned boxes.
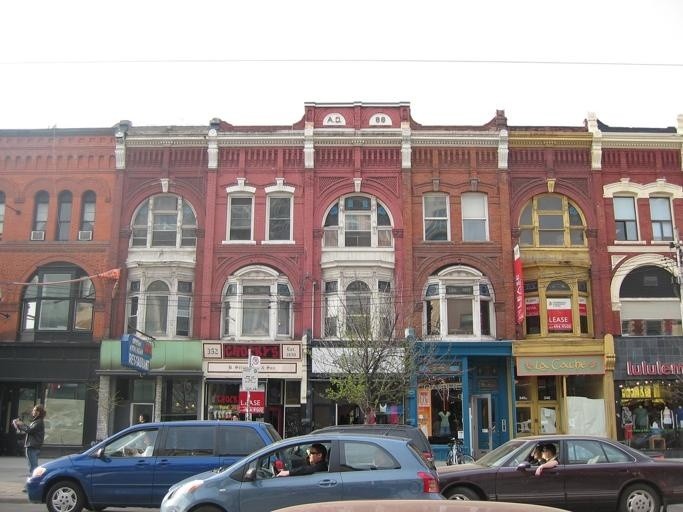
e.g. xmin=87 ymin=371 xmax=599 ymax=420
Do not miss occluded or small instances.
xmin=27 ymin=421 xmax=283 ymax=510
xmin=160 ymin=433 xmax=446 ymax=510
xmin=435 ymin=434 xmax=681 ymax=510
xmin=307 ymin=423 xmax=437 ymax=476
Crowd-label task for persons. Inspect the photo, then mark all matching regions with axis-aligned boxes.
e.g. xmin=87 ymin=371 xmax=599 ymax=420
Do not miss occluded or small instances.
xmin=285 ymin=443 xmax=309 ymax=468
xmin=438 ymin=408 xmax=452 ymax=433
xmin=534 ymin=443 xmax=559 ymax=476
xmin=527 ymin=443 xmax=544 ymax=466
xmin=12 ymin=403 xmax=46 ymax=492
xmin=624 ymin=420 xmax=633 ymax=447
xmin=622 ymin=402 xmax=682 ymax=430
xmin=129 ymin=432 xmax=155 ymax=457
xmin=138 ymin=412 xmax=149 ymax=423
xmin=274 ymin=444 xmax=327 ymax=477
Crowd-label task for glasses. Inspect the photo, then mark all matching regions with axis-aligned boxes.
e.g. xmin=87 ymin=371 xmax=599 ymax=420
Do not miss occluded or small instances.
xmin=309 ymin=451 xmax=316 ymax=456
